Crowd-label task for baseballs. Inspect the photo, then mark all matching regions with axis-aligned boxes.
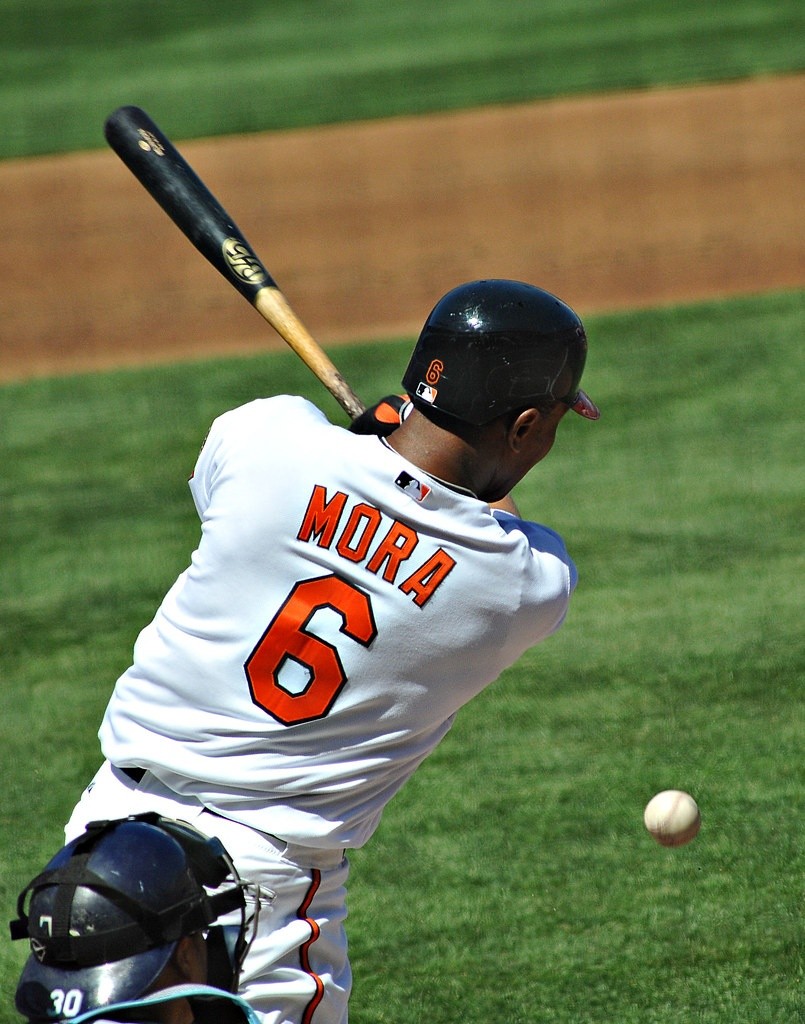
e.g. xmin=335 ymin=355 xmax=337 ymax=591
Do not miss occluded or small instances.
xmin=643 ymin=788 xmax=698 ymax=846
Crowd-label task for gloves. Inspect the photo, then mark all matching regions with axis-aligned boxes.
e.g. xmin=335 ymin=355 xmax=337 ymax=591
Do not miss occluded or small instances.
xmin=348 ymin=393 xmax=414 ymax=440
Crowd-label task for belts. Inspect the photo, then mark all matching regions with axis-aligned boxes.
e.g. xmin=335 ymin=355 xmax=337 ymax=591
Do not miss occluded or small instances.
xmin=120 ymin=767 xmax=282 ymax=843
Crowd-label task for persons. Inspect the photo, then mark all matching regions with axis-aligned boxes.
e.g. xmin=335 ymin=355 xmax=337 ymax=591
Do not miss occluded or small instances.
xmin=10 ymin=813 xmax=261 ymax=1024
xmin=65 ymin=279 xmax=599 ymax=1023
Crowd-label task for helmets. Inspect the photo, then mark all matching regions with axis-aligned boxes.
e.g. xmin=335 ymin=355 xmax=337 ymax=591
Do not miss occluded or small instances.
xmin=15 ymin=824 xmax=206 ymax=1024
xmin=401 ymin=279 xmax=601 ymax=425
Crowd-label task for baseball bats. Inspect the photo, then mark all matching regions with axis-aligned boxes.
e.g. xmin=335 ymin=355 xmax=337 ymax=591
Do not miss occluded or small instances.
xmin=101 ymin=103 xmax=376 ymax=432
